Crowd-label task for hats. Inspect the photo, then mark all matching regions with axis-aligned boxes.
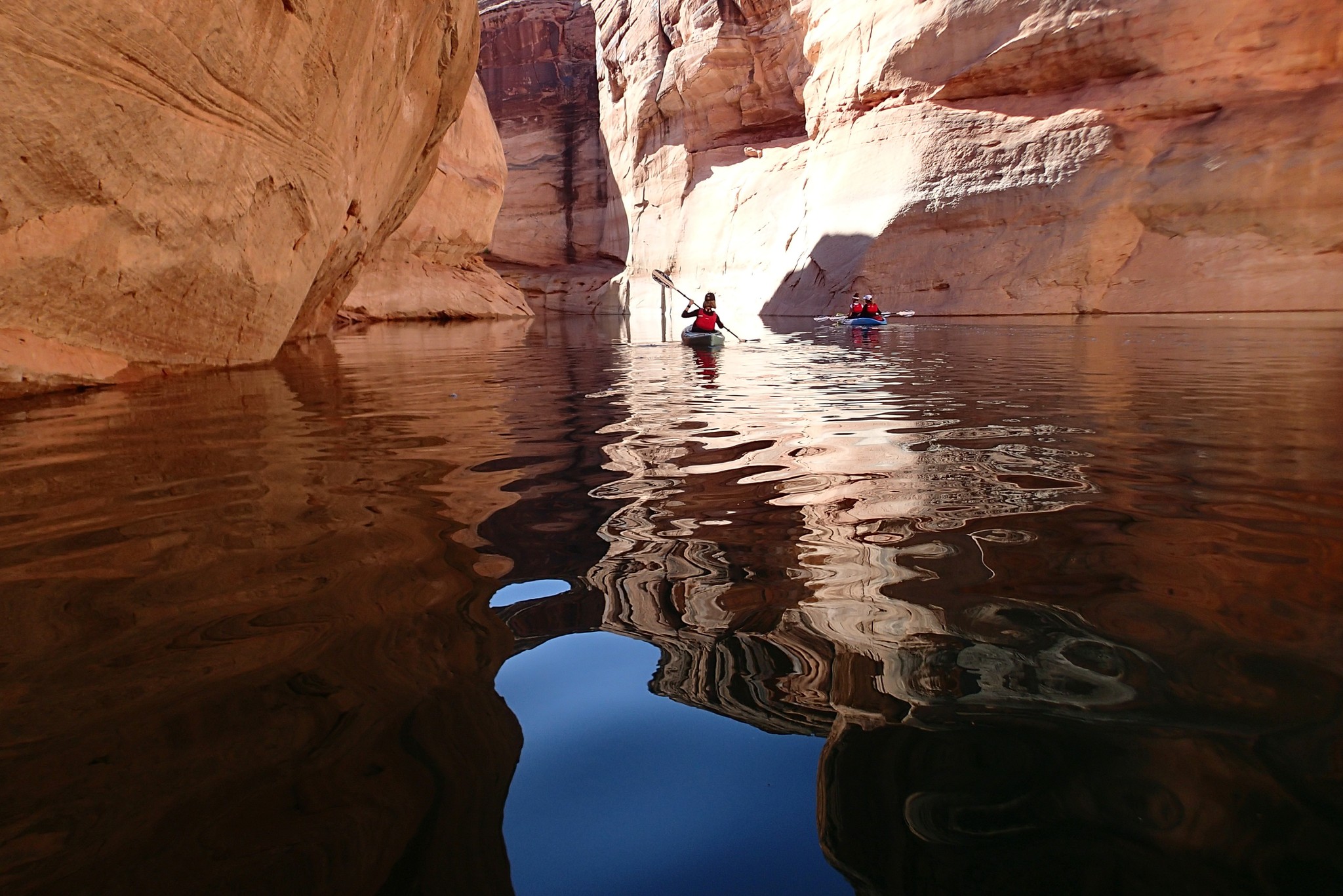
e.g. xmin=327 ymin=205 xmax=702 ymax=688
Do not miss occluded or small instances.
xmin=863 ymin=295 xmax=872 ymax=300
xmin=705 ymin=293 xmax=715 ymax=301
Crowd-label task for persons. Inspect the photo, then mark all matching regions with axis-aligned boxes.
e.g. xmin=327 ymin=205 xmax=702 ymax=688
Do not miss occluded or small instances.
xmin=844 ymin=293 xmax=882 ymax=319
xmin=681 ymin=293 xmax=723 ymax=334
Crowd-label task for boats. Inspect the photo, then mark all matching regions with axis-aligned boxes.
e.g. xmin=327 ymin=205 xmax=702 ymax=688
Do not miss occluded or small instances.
xmin=681 ymin=323 xmax=725 ymax=348
xmin=836 ymin=313 xmax=888 ymax=326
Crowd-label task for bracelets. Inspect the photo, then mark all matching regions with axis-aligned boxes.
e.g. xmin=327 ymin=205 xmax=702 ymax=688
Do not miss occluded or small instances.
xmin=688 ymin=305 xmax=690 ymax=308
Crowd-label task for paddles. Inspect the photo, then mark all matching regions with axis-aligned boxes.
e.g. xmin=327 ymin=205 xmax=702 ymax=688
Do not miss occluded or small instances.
xmin=813 ymin=311 xmax=890 ymax=323
xmin=829 ymin=310 xmax=916 ymax=322
xmin=651 ymin=269 xmax=747 ymax=343
